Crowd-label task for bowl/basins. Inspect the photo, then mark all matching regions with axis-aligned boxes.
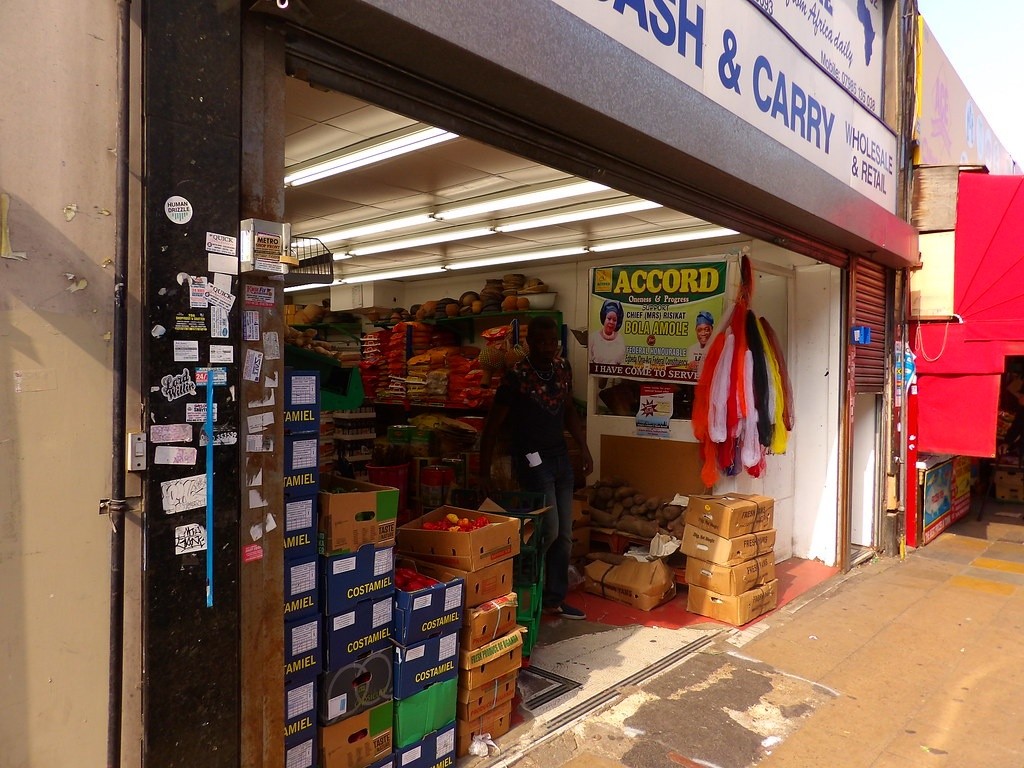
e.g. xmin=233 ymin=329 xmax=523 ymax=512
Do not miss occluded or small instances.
xmin=516 ymin=292 xmax=558 ymax=310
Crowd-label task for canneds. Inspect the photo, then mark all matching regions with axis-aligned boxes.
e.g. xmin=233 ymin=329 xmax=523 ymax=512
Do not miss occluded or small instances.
xmin=334 ymin=406 xmax=375 ymax=435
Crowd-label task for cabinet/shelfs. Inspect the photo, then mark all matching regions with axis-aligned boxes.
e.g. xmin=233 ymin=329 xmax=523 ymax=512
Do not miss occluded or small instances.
xmin=292 ymin=309 xmax=568 ymax=410
xmin=332 ymin=412 xmax=375 ymax=482
xmin=617 ymin=535 xmax=689 ymax=586
xmin=589 ymin=530 xmax=617 ymax=557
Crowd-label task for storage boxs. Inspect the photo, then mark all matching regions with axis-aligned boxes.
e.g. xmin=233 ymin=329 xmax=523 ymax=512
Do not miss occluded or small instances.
xmin=284 ymin=304 xmax=307 ymax=324
xmin=994 ymin=469 xmax=1024 ymax=503
xmin=282 ymin=335 xmax=779 ymax=768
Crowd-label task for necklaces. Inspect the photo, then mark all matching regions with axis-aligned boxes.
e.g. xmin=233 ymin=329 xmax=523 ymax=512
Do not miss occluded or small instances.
xmin=526 ymin=356 xmax=554 ymax=381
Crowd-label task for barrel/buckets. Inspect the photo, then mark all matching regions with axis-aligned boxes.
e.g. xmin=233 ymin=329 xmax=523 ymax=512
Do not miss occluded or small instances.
xmin=366 ymin=463 xmax=408 ymax=513
xmin=421 ymin=466 xmax=455 ymax=506
xmin=443 ymin=459 xmax=464 ymax=489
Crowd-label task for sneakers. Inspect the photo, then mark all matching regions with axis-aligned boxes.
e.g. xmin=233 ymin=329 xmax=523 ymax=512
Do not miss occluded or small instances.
xmin=554 ymin=603 xmax=586 ymax=620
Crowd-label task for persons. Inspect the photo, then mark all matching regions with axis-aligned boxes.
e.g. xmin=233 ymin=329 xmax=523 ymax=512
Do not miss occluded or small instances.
xmin=590 ymin=300 xmax=626 ymax=365
xmin=480 ymin=315 xmax=594 ymax=620
xmin=687 ymin=311 xmax=714 ymax=371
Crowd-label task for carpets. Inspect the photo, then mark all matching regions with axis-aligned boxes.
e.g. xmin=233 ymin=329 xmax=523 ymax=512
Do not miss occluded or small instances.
xmin=509 ymin=556 xmax=840 ymax=729
